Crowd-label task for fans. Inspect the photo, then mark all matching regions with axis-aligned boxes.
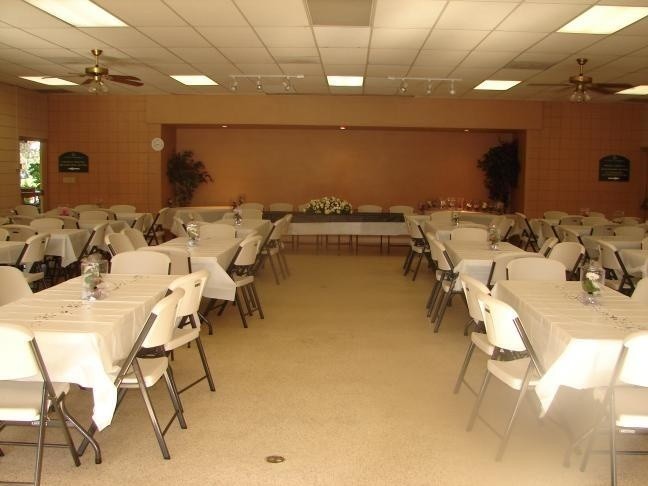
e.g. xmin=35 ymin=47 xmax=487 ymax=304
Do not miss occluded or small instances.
xmin=41 ymin=48 xmax=145 ymax=88
xmin=525 ymin=56 xmax=634 ymax=96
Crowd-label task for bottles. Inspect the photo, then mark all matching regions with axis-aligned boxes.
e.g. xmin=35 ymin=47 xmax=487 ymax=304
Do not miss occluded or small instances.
xmin=187 ymin=217 xmax=200 ymax=248
xmin=448 ymin=208 xmax=460 ymax=227
xmin=579 ymin=247 xmax=606 ymax=308
xmin=486 ymin=222 xmax=500 ymax=250
xmin=80 ymin=258 xmax=110 ymax=300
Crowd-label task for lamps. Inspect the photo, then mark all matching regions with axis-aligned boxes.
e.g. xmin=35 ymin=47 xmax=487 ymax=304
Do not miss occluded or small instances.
xmin=226 ymin=75 xmax=306 ymax=95
xmin=81 ymin=75 xmax=111 ymax=96
xmin=566 ymin=86 xmax=591 ymax=105
xmin=386 ymin=76 xmax=463 ymax=97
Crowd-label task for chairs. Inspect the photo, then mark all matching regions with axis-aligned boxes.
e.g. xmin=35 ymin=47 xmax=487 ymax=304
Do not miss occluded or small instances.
xmin=404 ymin=197 xmax=647 ymax=484
xmin=296 ymin=205 xmax=414 ymax=258
xmin=0 ymin=201 xmax=296 ymax=484
xmin=19 ymin=186 xmax=40 ymax=206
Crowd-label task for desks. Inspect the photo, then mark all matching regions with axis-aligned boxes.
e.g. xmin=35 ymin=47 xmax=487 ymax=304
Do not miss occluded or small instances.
xmin=22 ymin=191 xmax=40 ymax=196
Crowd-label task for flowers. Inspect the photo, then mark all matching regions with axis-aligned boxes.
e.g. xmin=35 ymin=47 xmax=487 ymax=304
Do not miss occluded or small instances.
xmin=301 ymin=195 xmax=354 ymax=216
